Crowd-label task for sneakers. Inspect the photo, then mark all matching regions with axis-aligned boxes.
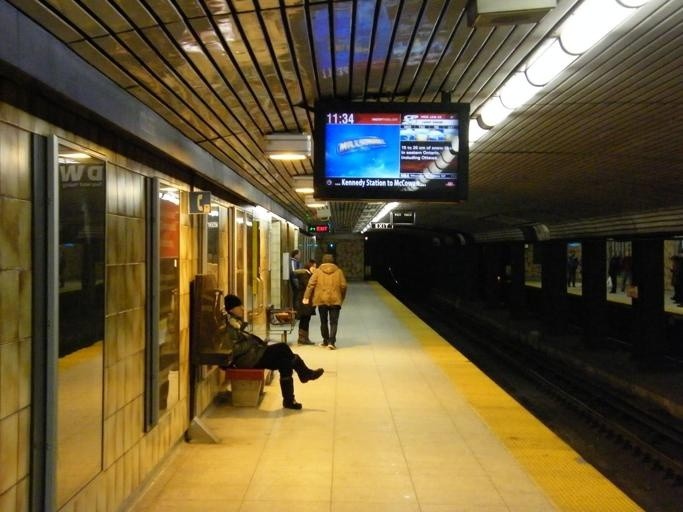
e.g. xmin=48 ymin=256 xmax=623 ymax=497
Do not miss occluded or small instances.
xmin=319 ymin=342 xmax=336 ymax=349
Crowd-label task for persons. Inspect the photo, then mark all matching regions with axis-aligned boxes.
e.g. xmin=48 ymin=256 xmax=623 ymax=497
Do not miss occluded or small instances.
xmin=300 ymin=252 xmax=347 ymax=350
xmin=221 ymin=295 xmax=324 ymax=409
xmin=293 ymin=259 xmax=318 ymax=345
xmin=287 ymin=249 xmax=302 ymax=305
xmin=566 ymin=250 xmax=683 ymax=310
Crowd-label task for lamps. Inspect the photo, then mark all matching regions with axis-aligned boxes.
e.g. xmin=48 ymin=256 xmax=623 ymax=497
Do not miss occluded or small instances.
xmin=264 ymin=132 xmax=329 ymax=211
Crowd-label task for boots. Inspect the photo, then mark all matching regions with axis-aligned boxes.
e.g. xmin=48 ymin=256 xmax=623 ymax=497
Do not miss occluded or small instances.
xmin=292 ymin=355 xmax=323 ymax=383
xmin=281 ymin=377 xmax=302 ymax=409
xmin=298 ymin=329 xmax=314 ymax=344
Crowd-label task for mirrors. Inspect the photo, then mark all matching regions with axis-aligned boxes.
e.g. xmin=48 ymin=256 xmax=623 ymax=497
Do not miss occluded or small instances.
xmin=48 ymin=132 xmax=110 ymax=511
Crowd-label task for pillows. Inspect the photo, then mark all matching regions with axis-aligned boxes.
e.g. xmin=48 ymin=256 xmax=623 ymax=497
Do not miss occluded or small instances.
xmin=144 ymin=175 xmax=263 ymax=436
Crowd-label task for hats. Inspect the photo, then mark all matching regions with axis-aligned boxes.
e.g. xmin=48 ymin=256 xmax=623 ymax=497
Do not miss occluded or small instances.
xmin=225 ymin=295 xmax=241 ymax=310
xmin=322 ymin=254 xmax=333 ymax=263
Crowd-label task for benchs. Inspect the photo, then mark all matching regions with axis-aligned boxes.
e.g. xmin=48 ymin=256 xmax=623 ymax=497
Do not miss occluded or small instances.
xmin=221 ymin=303 xmax=298 ymax=408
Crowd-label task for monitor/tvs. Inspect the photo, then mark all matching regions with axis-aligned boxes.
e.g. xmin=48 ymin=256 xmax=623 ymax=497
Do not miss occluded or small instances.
xmin=313 ymin=99 xmax=470 ymax=202
